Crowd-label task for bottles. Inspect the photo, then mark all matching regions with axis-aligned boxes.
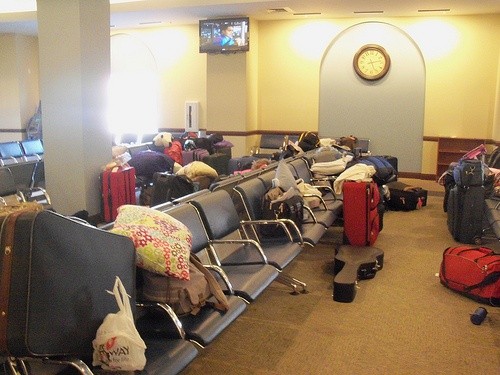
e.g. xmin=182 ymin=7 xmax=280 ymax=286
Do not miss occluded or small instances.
xmin=198 ymin=129 xmax=206 ymax=138
xmin=471 ymin=307 xmax=487 ymax=325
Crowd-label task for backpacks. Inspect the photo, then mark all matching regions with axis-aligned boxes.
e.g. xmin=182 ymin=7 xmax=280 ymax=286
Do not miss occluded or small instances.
xmin=488 ymin=145 xmax=500 ymax=169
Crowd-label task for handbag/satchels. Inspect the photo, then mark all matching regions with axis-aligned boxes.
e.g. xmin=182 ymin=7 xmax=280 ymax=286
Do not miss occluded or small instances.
xmin=382 ymin=180 xmax=428 ymax=212
xmin=452 ymin=158 xmax=486 ymax=187
xmin=0 ymin=204 xmax=136 ymax=360
xmin=439 ymin=244 xmax=500 ymax=307
xmin=258 ymin=181 xmax=305 ymax=239
xmin=138 ymin=130 xmax=262 ymax=208
xmin=134 ymin=250 xmax=231 ymax=321
xmin=93 ymin=275 xmax=148 ymax=373
xmin=270 ymin=130 xmax=399 ymax=188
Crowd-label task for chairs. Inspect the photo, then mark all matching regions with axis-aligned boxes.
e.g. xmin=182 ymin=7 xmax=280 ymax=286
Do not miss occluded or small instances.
xmin=477 ymin=144 xmax=500 ymax=244
xmin=0 ymin=135 xmax=371 ymax=375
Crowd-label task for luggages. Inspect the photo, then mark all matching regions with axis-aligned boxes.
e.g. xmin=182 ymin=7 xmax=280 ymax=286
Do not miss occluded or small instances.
xmin=99 ymin=162 xmax=137 ymax=224
xmin=447 ymin=184 xmax=487 ymax=245
xmin=341 ymin=177 xmax=382 ymax=247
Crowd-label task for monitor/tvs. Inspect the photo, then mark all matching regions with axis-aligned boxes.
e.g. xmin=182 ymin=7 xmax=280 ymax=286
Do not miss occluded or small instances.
xmin=0 ymin=141 xmax=23 ymax=159
xmin=199 ymin=16 xmax=250 ymax=52
xmin=20 ymin=138 xmax=44 ymax=155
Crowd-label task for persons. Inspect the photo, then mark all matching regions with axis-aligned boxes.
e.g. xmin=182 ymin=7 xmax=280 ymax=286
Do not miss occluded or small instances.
xmin=217 ymin=24 xmax=235 ymax=47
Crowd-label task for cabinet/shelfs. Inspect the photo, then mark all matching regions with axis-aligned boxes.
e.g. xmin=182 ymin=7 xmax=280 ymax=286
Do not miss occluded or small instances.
xmin=436 ymin=137 xmax=493 ymax=184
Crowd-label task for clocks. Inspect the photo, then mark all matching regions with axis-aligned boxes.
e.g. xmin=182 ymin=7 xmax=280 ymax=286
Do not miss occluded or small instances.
xmin=353 ymin=44 xmax=391 ymax=80
